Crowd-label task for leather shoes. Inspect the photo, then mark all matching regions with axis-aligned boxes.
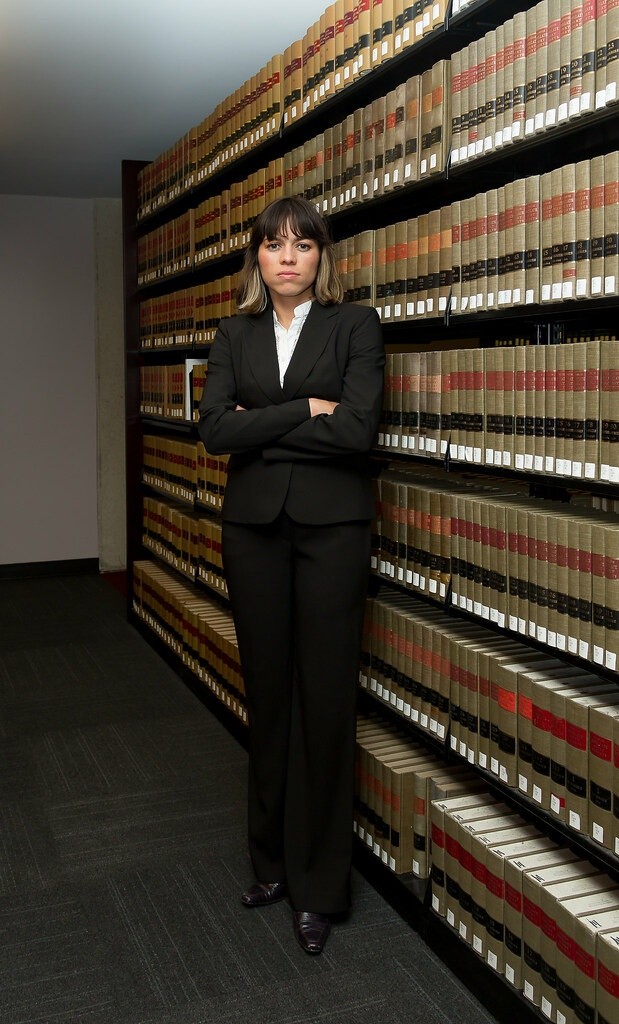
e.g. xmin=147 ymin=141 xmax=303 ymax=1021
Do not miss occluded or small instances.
xmin=240 ymin=880 xmax=289 ymax=906
xmin=294 ymin=909 xmax=332 ymax=953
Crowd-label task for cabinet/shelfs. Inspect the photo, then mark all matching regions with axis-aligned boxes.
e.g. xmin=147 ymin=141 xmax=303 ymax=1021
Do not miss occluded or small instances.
xmin=123 ymin=0 xmax=618 ymax=1024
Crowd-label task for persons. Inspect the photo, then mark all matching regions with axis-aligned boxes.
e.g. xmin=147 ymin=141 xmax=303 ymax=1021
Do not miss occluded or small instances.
xmin=200 ymin=197 xmax=388 ymax=955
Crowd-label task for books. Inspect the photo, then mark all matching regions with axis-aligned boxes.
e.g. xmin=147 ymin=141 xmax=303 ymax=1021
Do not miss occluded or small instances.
xmin=134 ymin=1 xmax=617 ymax=1024
